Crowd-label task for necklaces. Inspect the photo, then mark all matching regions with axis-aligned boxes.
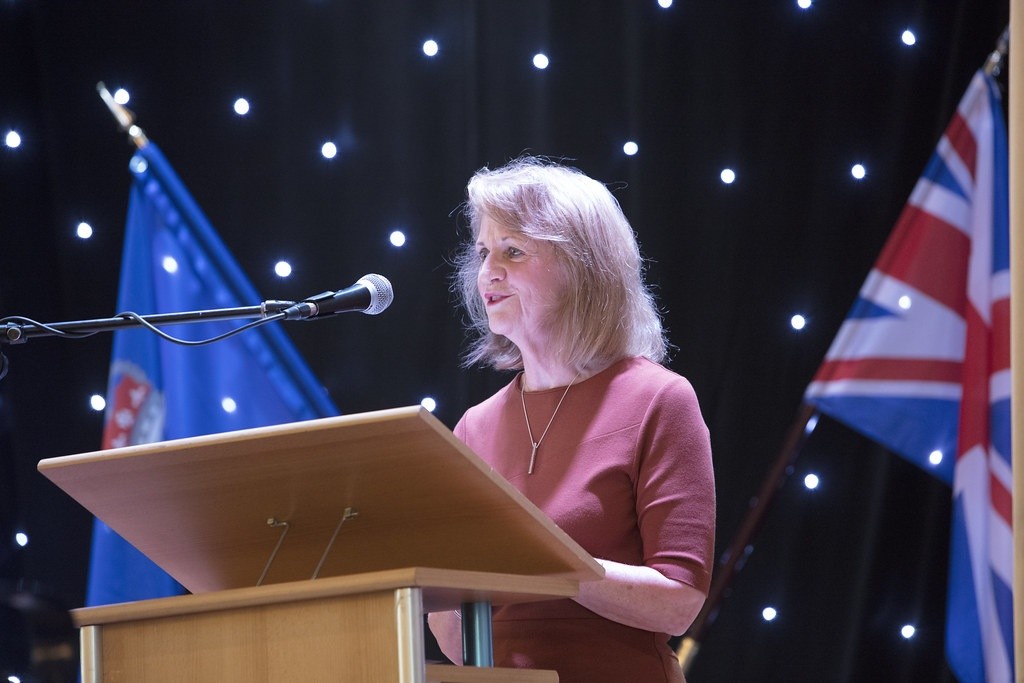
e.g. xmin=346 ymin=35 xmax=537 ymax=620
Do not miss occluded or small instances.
xmin=521 ymin=370 xmax=584 ymax=475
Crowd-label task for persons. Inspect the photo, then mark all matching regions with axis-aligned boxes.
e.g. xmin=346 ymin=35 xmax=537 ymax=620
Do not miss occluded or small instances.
xmin=428 ymin=156 xmax=716 ymax=683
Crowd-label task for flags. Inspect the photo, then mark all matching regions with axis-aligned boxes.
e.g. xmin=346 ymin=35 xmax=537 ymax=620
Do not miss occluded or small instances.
xmin=84 ymin=139 xmax=342 ymax=610
xmin=804 ymin=67 xmax=1018 ymax=683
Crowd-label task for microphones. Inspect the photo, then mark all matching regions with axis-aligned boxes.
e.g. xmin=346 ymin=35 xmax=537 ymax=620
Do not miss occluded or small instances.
xmin=283 ymin=273 xmax=395 ymax=320
